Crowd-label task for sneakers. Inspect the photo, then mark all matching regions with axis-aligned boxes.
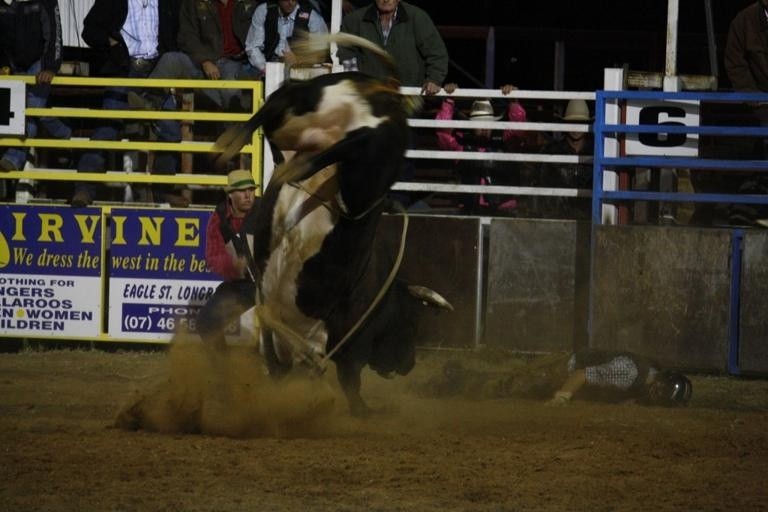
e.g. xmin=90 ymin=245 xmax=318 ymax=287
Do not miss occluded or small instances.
xmin=158 ymin=193 xmax=189 ymax=207
xmin=0 ymin=158 xmax=17 ymax=201
xmin=72 ymin=189 xmax=91 ymax=208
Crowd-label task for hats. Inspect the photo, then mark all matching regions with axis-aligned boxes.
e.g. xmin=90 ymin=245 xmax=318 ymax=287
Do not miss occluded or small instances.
xmin=561 ymin=100 xmax=595 ymax=122
xmin=666 ymin=373 xmax=692 ymax=403
xmin=459 ymin=100 xmax=504 ymax=121
xmin=223 ymin=169 xmax=259 ymax=192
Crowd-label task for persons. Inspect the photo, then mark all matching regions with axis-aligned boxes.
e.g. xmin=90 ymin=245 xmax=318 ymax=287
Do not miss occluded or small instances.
xmin=436 ymin=82 xmax=593 ymax=220
xmin=338 ymin=2 xmax=449 ymax=209
xmin=423 ymin=346 xmax=693 ymax=410
xmin=0 ymin=1 xmax=261 ymax=206
xmin=245 ymin=2 xmax=330 ymax=75
xmin=196 ymin=169 xmax=290 ymax=383
xmin=724 ymin=1 xmax=767 ymax=196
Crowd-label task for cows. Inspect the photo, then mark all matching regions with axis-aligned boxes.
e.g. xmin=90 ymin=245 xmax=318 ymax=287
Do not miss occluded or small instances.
xmin=117 ymin=28 xmax=456 ymax=432
